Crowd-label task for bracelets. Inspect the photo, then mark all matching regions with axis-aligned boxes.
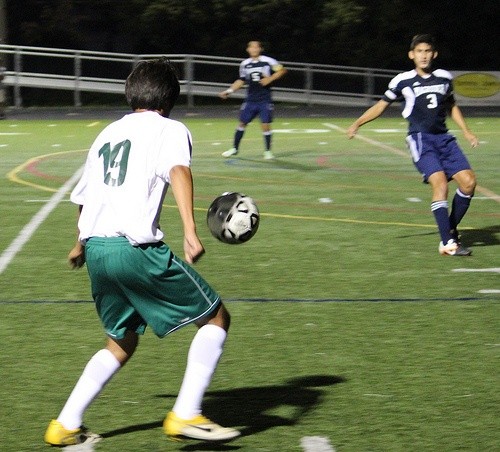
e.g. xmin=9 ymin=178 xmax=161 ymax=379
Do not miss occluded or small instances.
xmin=226 ymin=89 xmax=235 ymax=95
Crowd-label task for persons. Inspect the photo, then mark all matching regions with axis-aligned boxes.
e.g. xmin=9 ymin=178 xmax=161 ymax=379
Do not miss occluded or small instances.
xmin=221 ymin=38 xmax=287 ymax=161
xmin=347 ymin=32 xmax=479 ymax=256
xmin=44 ymin=56 xmax=241 ymax=447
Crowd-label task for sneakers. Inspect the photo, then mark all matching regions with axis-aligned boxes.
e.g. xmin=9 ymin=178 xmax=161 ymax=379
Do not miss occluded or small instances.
xmin=439 ymin=239 xmax=472 ymax=256
xmin=45 ymin=420 xmax=102 ymax=445
xmin=163 ymin=412 xmax=241 ymax=440
xmin=223 ymin=148 xmax=238 ymax=157
xmin=453 ymin=232 xmax=464 ymax=245
xmin=264 ymin=151 xmax=273 ymax=159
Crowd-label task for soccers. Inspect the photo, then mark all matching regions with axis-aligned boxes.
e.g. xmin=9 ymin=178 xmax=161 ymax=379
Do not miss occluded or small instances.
xmin=207 ymin=191 xmax=260 ymax=245
